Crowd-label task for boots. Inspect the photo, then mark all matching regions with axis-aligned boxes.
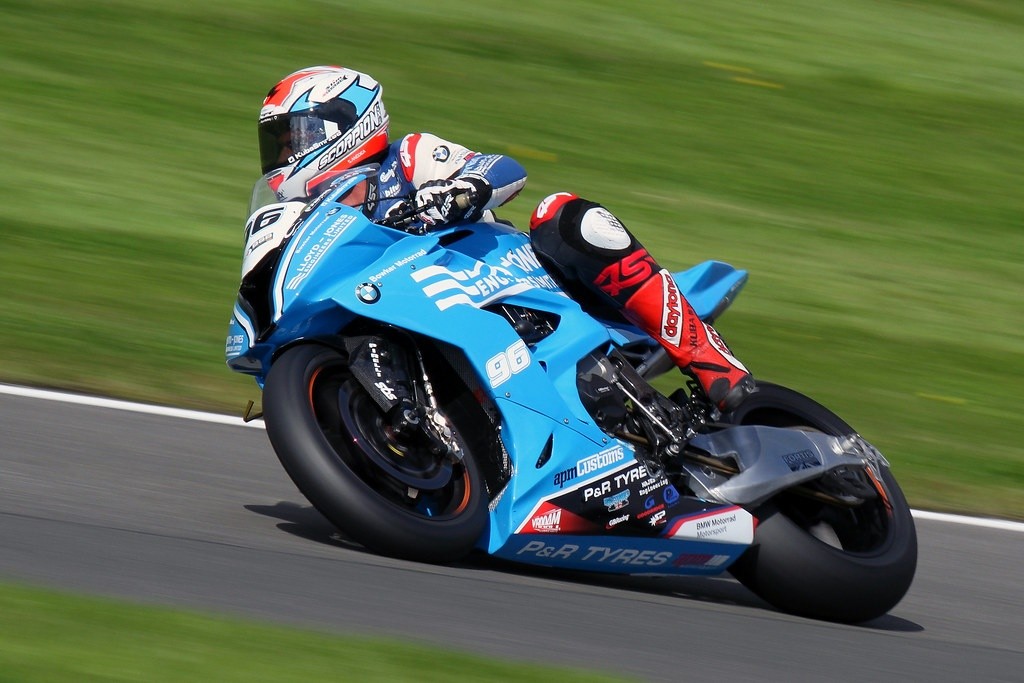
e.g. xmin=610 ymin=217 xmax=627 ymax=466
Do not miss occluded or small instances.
xmin=620 ymin=268 xmax=753 ymax=414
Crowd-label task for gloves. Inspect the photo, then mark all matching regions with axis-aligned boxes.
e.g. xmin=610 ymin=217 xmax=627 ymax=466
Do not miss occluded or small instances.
xmin=411 ymin=177 xmax=487 ymax=225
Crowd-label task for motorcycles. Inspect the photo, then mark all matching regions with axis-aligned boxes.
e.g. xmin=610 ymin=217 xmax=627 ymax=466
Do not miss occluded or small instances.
xmin=222 ymin=162 xmax=919 ymax=624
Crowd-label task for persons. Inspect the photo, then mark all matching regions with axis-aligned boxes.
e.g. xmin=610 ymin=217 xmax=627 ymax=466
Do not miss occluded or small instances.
xmin=257 ymin=65 xmax=760 ymax=412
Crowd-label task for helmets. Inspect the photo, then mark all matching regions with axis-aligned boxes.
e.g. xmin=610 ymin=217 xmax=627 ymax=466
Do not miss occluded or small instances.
xmin=256 ymin=66 xmax=389 ymax=202
